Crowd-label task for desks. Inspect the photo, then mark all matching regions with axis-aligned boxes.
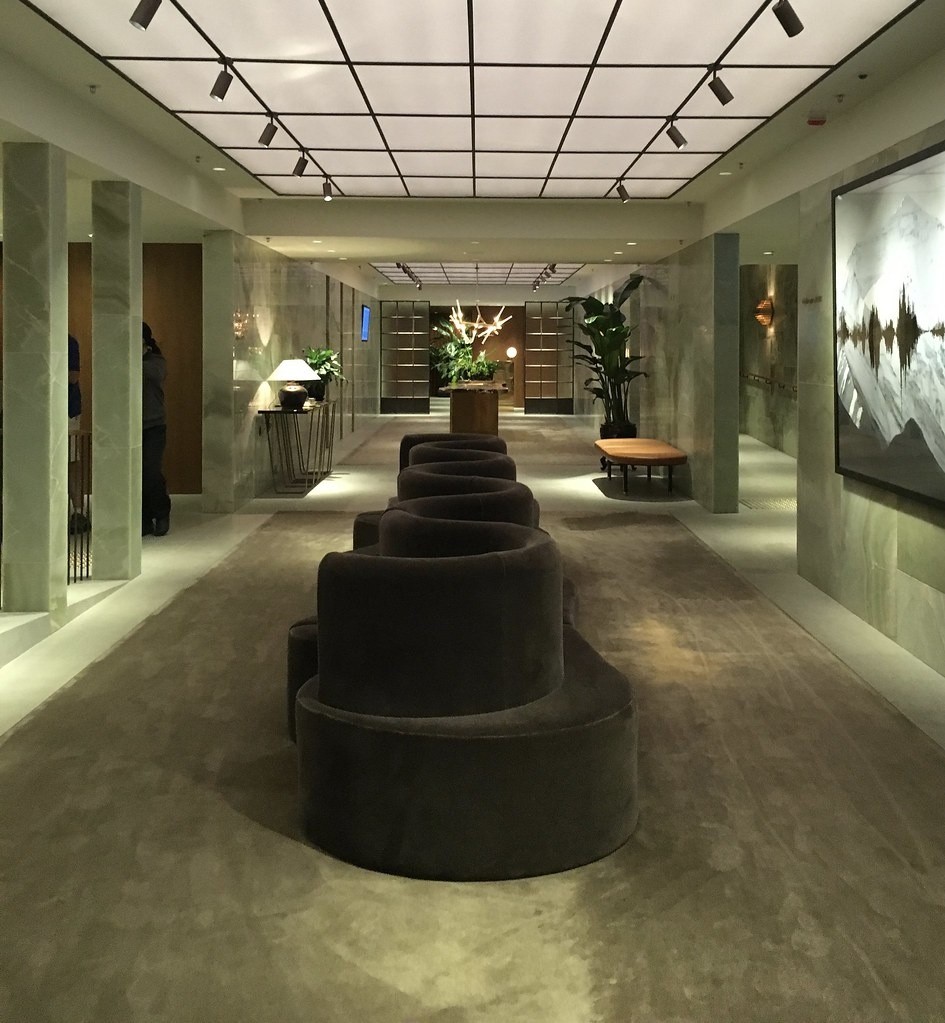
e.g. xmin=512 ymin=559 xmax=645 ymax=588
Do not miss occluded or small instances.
xmin=257 ymin=398 xmax=339 ymax=495
xmin=595 ymin=438 xmax=686 ymax=497
xmin=440 ymin=380 xmax=509 ymax=435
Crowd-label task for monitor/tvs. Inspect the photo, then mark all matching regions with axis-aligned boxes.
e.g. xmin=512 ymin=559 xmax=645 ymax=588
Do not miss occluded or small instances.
xmin=361 ymin=304 xmax=370 ymax=341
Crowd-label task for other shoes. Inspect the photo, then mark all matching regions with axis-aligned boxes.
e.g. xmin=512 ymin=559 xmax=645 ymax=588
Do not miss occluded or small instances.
xmin=154 ymin=514 xmax=169 ymax=536
xmin=70 ymin=513 xmax=91 ymax=536
xmin=142 ymin=520 xmax=154 ymax=536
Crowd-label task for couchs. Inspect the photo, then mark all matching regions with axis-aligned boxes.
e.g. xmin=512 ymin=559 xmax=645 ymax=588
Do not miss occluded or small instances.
xmin=286 ymin=432 xmax=639 ymax=882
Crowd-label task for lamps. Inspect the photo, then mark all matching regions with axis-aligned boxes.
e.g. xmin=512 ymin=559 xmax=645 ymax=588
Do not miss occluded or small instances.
xmin=209 ymin=64 xmax=233 ymax=101
xmin=544 ymin=268 xmax=551 ymax=278
xmin=322 ymin=176 xmax=333 ymax=201
xmin=772 ymin=0 xmax=804 ymax=38
xmin=533 ymin=279 xmax=540 ymax=292
xmin=129 ymin=0 xmax=163 ymax=31
xmin=292 ymin=150 xmax=308 ymax=178
xmin=396 ymin=262 xmax=422 ymax=290
xmin=549 ymin=264 xmax=556 ymax=273
xmin=616 ymin=178 xmax=630 ymax=203
xmin=268 ymin=359 xmax=321 ymax=411
xmin=755 ymin=299 xmax=772 ymax=327
xmin=258 ymin=118 xmax=279 ymax=147
xmin=540 ymin=274 xmax=547 ymax=282
xmin=666 ymin=115 xmax=688 ymax=150
xmin=706 ymin=63 xmax=734 ymax=106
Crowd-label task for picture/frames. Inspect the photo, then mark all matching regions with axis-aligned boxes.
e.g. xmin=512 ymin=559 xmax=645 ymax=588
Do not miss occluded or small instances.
xmin=830 ymin=141 xmax=944 ymax=508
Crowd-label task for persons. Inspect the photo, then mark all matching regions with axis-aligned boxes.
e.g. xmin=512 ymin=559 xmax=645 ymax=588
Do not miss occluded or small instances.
xmin=143 ymin=321 xmax=172 ymax=536
xmin=68 ymin=334 xmax=93 ymax=535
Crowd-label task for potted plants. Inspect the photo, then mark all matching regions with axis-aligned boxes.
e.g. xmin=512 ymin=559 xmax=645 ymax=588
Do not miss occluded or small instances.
xmin=559 ymin=274 xmax=649 ymax=470
xmin=299 ymin=346 xmax=350 ymax=400
